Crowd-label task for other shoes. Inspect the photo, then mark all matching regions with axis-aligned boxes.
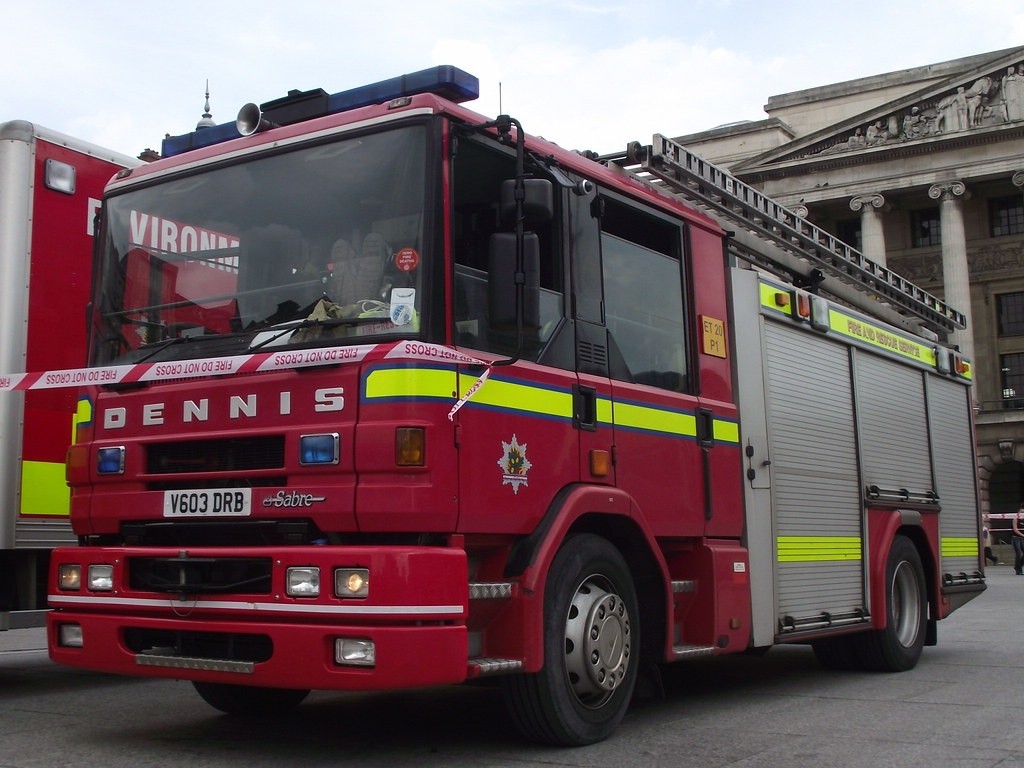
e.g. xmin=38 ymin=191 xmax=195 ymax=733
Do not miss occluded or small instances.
xmin=331 ymin=238 xmax=355 ymax=303
xmin=355 ymin=231 xmax=383 ymax=299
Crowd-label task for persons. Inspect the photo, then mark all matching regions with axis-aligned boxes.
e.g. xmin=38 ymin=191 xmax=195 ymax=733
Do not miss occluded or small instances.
xmin=982 ymin=520 xmax=998 ymax=566
xmin=849 ymin=64 xmax=1024 ymax=149
xmin=1012 ymin=506 xmax=1024 ymax=575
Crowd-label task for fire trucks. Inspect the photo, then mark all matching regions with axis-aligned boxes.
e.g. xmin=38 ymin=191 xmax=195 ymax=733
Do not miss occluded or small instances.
xmin=0 ymin=120 xmax=239 ymax=643
xmin=49 ymin=63 xmax=989 ymax=747
